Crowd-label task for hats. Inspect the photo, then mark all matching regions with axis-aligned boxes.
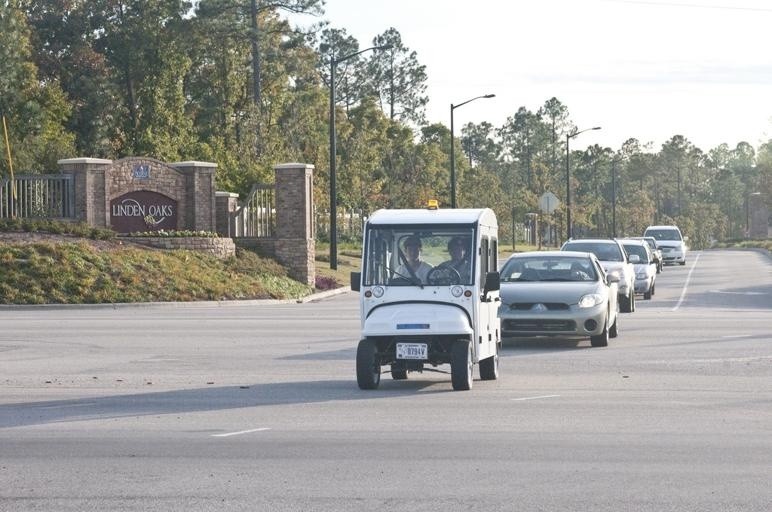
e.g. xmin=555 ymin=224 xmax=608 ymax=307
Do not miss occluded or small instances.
xmin=403 ymin=237 xmax=421 ymax=249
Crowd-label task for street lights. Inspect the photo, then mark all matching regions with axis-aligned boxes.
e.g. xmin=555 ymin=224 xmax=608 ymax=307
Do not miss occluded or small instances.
xmin=327 ymin=40 xmax=398 ymax=270
xmin=563 ymin=124 xmax=603 ymax=242
xmin=610 ymin=153 xmax=642 ymax=240
xmin=448 ymin=91 xmax=497 ymax=207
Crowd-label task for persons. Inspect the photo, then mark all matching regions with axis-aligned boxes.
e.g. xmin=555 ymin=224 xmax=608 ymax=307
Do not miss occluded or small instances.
xmin=390 ymin=235 xmax=435 ymax=284
xmin=432 ymin=236 xmax=471 ymax=285
xmin=568 ymin=260 xmax=592 ymax=281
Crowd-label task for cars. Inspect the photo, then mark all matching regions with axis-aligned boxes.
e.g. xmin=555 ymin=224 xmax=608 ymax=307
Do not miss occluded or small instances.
xmin=498 ymin=234 xmax=663 ymax=348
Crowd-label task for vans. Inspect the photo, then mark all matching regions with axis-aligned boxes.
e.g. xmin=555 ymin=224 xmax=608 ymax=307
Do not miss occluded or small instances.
xmin=644 ymin=223 xmax=689 ymax=266
